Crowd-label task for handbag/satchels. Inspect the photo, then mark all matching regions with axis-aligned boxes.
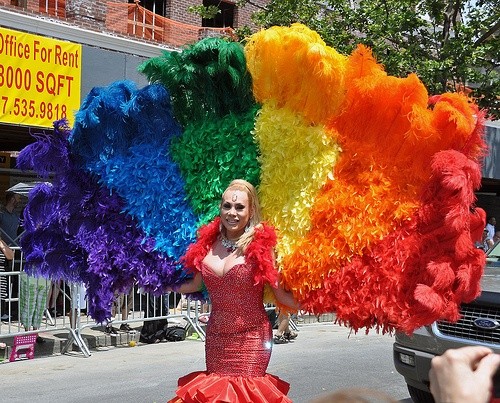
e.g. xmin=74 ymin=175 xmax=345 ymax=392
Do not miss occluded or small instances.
xmin=165 ymin=325 xmax=186 ymax=342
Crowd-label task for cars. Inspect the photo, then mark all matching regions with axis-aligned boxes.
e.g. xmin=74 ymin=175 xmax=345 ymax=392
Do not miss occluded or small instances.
xmin=393 ymin=240 xmax=500 ymax=403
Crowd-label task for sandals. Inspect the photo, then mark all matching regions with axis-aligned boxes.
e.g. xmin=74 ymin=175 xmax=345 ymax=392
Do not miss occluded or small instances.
xmin=284 ymin=331 xmax=298 ymax=340
xmin=274 ymin=334 xmax=289 ymax=344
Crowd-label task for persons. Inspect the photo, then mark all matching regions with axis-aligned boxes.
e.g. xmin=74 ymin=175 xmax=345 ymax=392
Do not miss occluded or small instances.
xmin=20 ymin=269 xmax=52 ymax=344
xmin=104 ymin=287 xmax=136 ymax=336
xmin=273 ymin=308 xmax=299 ymax=344
xmin=0 ymin=192 xmax=22 ymax=321
xmin=67 ymin=282 xmax=87 ymax=347
xmin=166 ymin=179 xmax=304 ymax=403
xmin=139 ymin=292 xmax=169 ymax=344
xmin=476 ymin=216 xmax=500 ymax=253
xmin=42 ymin=279 xmax=61 ymax=320
xmin=428 ymin=345 xmax=500 ymax=403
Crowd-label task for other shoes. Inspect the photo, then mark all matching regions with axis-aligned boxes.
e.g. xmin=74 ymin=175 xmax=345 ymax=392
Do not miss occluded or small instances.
xmin=105 ymin=322 xmax=137 ymax=335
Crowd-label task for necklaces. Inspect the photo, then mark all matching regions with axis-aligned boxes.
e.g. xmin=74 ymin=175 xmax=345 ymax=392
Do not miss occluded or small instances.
xmin=220 ymin=232 xmax=243 ymax=253
